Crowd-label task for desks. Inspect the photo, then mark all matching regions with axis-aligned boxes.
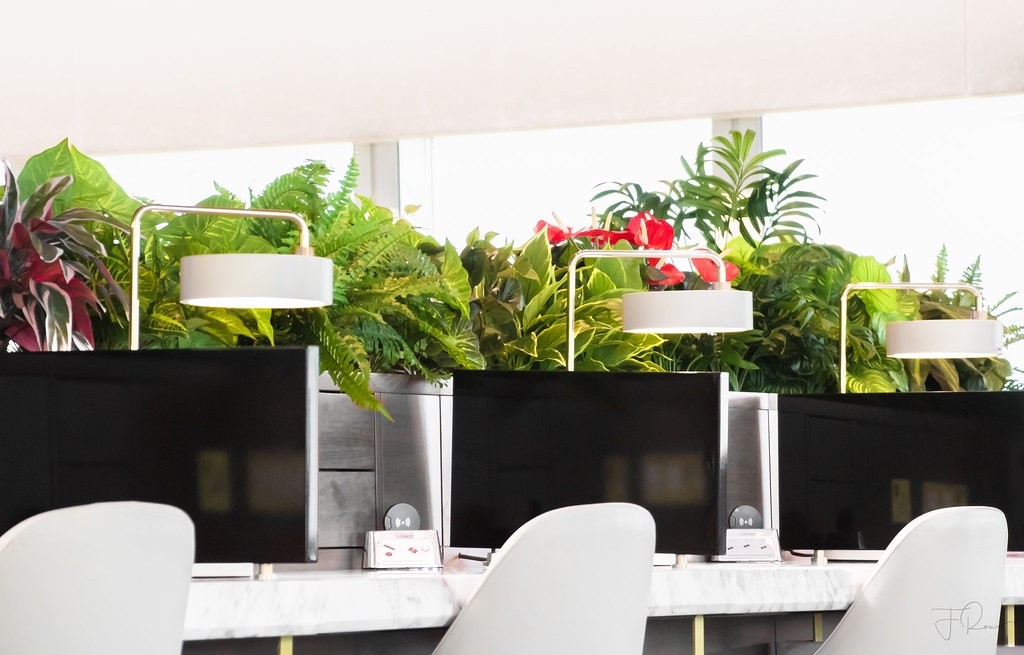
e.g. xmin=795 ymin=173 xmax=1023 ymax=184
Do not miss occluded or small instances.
xmin=0 ymin=345 xmax=1024 ymax=655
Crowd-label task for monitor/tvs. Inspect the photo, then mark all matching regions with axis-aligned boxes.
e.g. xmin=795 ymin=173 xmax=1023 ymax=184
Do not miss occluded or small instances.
xmin=449 ymin=369 xmax=729 ymax=565
xmin=0 ymin=346 xmax=320 ymax=579
xmin=777 ymin=394 xmax=1024 ymax=560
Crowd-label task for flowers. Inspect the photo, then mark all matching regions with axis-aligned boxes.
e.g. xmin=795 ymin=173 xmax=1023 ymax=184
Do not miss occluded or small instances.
xmin=534 ymin=206 xmax=740 ymax=289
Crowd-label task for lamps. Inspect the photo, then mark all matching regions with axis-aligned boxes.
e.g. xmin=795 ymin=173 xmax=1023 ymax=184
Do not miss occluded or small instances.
xmin=565 ymin=249 xmax=754 ymax=372
xmin=840 ymin=283 xmax=1004 ymax=394
xmin=130 ymin=206 xmax=332 ymax=352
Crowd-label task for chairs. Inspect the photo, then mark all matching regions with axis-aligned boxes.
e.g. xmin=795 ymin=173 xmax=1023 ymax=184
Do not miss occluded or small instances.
xmin=0 ymin=499 xmax=195 ymax=655
xmin=429 ymin=501 xmax=656 ymax=654
xmin=810 ymin=506 xmax=1008 ymax=654
